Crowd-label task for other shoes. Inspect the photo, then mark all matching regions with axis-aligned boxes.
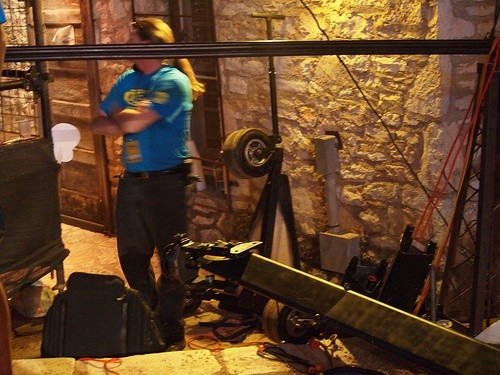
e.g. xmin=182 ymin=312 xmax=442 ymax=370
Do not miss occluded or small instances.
xmin=153 ymin=318 xmax=186 ymax=342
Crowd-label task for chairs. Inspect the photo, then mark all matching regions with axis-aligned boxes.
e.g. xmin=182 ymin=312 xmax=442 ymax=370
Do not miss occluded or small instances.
xmin=0 ymin=138 xmax=73 ymax=375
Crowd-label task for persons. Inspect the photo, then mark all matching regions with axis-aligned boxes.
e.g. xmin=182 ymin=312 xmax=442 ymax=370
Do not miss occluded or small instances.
xmin=90 ymin=18 xmax=206 ymax=354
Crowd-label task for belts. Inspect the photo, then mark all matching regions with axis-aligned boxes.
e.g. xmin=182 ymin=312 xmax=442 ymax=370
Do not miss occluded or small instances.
xmin=126 ymin=167 xmax=179 ymax=179
